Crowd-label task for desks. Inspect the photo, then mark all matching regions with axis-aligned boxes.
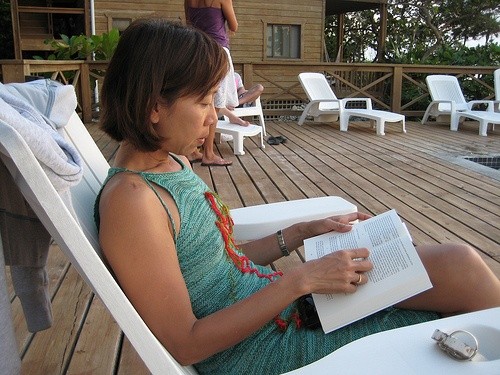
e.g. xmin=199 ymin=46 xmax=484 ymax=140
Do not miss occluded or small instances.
xmin=0 ymin=59 xmax=110 ymax=124
xmin=281 ymin=306 xmax=500 ymax=375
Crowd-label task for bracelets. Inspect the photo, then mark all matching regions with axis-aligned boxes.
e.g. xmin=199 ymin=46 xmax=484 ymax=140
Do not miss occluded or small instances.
xmin=276 ymin=229 xmax=290 ymax=257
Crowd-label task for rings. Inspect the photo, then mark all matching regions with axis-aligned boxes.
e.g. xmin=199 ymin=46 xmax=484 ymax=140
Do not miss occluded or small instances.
xmin=356 ymin=274 xmax=362 ymax=284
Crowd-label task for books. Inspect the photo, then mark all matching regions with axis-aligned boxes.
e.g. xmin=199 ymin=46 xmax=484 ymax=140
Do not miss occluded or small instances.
xmin=302 ymin=209 xmax=433 ymax=335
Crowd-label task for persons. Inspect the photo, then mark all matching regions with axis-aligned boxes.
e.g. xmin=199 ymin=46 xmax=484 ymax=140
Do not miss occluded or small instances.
xmin=93 ymin=17 xmax=500 ymax=375
xmin=184 ymin=0 xmax=263 ymax=166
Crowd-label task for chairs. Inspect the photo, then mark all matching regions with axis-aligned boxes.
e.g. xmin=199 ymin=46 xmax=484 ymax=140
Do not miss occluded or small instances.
xmin=216 ymin=118 xmax=265 ymax=156
xmin=298 ymin=72 xmax=407 ymax=136
xmin=223 ymin=96 xmax=267 ymax=137
xmin=0 ymin=78 xmax=358 ymax=375
xmin=421 ymin=74 xmax=500 ymax=136
xmin=493 ymin=69 xmax=500 ymax=110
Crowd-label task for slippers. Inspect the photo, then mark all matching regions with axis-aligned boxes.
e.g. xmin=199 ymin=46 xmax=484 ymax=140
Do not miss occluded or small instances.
xmin=268 ymin=136 xmax=280 ymax=145
xmin=202 ymin=158 xmax=233 ymax=167
xmin=187 ymin=157 xmax=204 ymax=163
xmin=276 ymin=136 xmax=287 ymax=144
